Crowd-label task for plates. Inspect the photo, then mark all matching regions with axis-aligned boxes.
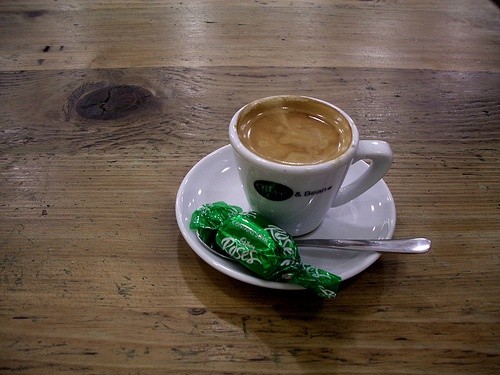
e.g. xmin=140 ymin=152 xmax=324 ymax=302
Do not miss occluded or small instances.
xmin=175 ymin=142 xmax=396 ymax=290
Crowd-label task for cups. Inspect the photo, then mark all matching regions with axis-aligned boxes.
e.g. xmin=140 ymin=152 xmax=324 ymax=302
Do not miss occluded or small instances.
xmin=228 ymin=95 xmax=394 ymax=239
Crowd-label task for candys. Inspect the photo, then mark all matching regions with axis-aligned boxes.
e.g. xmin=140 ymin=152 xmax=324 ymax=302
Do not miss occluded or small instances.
xmin=192 ymin=201 xmax=343 ymax=303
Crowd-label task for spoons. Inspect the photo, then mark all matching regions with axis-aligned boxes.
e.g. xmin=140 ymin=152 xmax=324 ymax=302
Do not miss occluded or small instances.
xmin=196 ymin=228 xmax=434 ymax=261
